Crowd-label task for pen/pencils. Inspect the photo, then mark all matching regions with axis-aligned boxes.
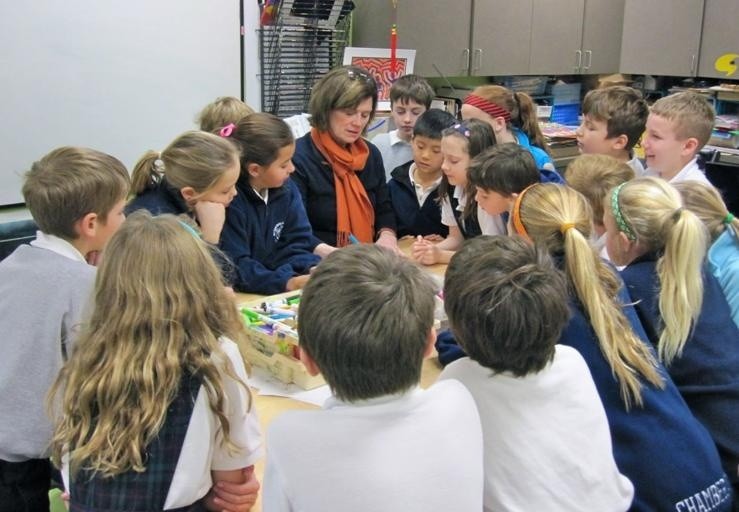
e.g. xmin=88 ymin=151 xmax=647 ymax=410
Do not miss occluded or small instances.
xmin=348 ymin=231 xmax=358 ymax=245
xmin=238 ymin=295 xmax=301 ymax=340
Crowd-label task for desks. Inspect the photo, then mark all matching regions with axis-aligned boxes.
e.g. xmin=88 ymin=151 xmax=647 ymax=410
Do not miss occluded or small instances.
xmin=234 ymin=238 xmax=460 ymax=512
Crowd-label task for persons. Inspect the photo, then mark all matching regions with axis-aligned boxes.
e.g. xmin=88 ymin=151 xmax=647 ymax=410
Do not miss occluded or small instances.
xmin=0 ymin=67 xmax=739 ymax=511
